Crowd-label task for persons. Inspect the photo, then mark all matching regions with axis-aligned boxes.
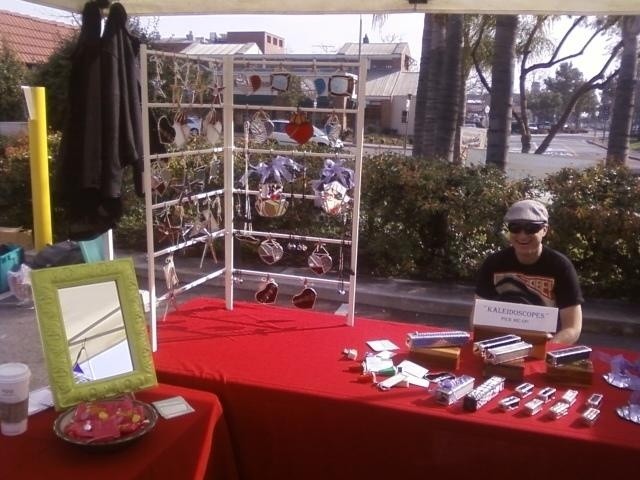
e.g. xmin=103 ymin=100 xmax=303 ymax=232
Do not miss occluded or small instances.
xmin=469 ymin=199 xmax=585 ymax=345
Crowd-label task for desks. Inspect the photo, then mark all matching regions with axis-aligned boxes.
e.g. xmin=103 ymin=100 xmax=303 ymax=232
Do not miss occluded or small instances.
xmin=0 ymin=382 xmax=242 ymax=480
xmin=145 ymin=299 xmax=639 ymax=480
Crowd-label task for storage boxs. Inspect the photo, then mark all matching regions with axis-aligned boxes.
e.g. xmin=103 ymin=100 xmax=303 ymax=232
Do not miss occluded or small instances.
xmin=405 ymin=315 xmax=604 ymax=426
xmin=1 ymin=244 xmax=20 ymax=293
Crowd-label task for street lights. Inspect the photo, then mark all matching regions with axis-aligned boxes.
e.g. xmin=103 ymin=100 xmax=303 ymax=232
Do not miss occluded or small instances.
xmin=594 ymin=111 xmax=599 ymax=137
xmin=485 ymin=106 xmax=490 ymax=128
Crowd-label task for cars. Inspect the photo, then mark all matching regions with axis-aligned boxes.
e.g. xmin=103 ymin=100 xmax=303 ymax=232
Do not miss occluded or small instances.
xmin=267 ymin=120 xmax=344 ymax=148
xmin=186 ymin=117 xmax=200 ymax=135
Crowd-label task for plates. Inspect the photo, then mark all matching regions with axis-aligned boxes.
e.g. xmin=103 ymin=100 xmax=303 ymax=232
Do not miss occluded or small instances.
xmin=51 ymin=399 xmax=161 ymax=448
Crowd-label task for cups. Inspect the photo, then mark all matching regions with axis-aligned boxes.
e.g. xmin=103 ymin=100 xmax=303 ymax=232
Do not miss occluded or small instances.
xmin=0 ymin=362 xmax=32 ymax=435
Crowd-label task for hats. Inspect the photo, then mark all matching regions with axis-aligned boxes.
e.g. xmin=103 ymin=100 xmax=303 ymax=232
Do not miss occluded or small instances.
xmin=504 ymin=195 xmax=550 ymax=224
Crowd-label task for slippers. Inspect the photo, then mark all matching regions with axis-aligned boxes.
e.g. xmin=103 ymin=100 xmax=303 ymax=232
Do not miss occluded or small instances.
xmin=506 ymin=220 xmax=545 ymax=236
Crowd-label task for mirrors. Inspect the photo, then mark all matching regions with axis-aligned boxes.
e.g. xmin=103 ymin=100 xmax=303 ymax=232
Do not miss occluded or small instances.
xmin=28 ymin=256 xmax=152 ymax=415
